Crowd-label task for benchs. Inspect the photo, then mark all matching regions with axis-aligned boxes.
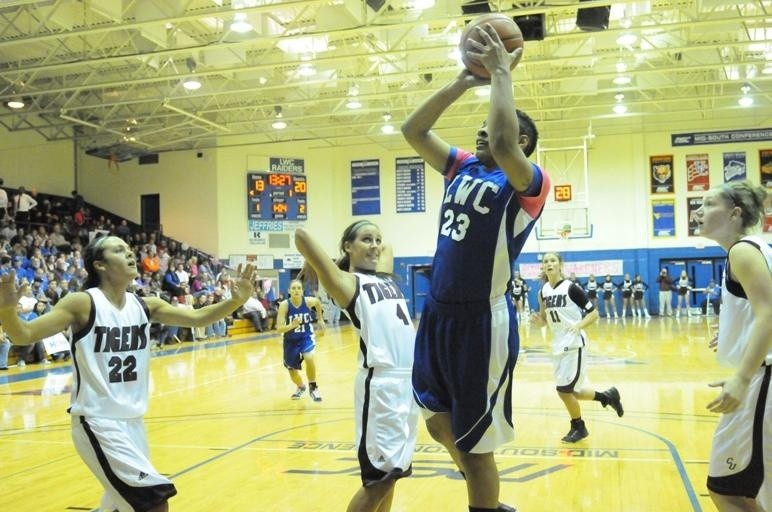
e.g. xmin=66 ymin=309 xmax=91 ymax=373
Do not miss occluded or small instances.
xmin=0 ymin=196 xmax=274 ymax=366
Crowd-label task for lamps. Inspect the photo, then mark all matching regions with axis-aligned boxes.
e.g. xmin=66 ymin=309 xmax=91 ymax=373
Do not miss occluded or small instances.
xmin=180 ymin=58 xmax=203 ymax=91
xmin=270 ymin=106 xmax=288 ymax=130
xmin=7 ymin=91 xmax=26 ymax=109
xmin=361 ymin=0 xmax=612 ymax=42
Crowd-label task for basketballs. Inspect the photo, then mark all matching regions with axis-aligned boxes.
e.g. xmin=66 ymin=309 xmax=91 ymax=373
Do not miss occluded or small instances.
xmin=460 ymin=14 xmax=524 ymax=79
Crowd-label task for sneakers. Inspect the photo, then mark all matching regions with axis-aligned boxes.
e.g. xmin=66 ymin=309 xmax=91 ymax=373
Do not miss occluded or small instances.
xmin=560 ymin=419 xmax=588 ymax=442
xmin=291 ymin=384 xmax=306 ymax=398
xmin=309 ymin=387 xmax=321 ymax=402
xmin=601 ymin=387 xmax=623 ymax=417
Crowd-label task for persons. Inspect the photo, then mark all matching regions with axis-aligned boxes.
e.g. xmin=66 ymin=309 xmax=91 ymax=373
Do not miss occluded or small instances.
xmin=0 ymin=235 xmax=258 ymax=512
xmin=324 ymin=296 xmax=341 ymax=324
xmin=694 ymin=180 xmax=771 ymax=512
xmin=398 ymin=23 xmax=550 ymax=512
xmin=93 ymin=214 xmax=278 ymax=348
xmin=656 ymin=267 xmax=673 ymax=316
xmin=294 ymin=219 xmax=421 ymax=512
xmin=567 ymin=272 xmax=651 ymax=321
xmin=530 ymin=252 xmax=624 ymax=443
xmin=701 ymin=279 xmax=721 ymax=317
xmin=509 ymin=270 xmax=529 ymax=328
xmin=276 ymin=279 xmax=325 ymax=401
xmin=0 ymin=179 xmax=92 ymax=370
xmin=675 ymin=270 xmax=693 ymax=317
